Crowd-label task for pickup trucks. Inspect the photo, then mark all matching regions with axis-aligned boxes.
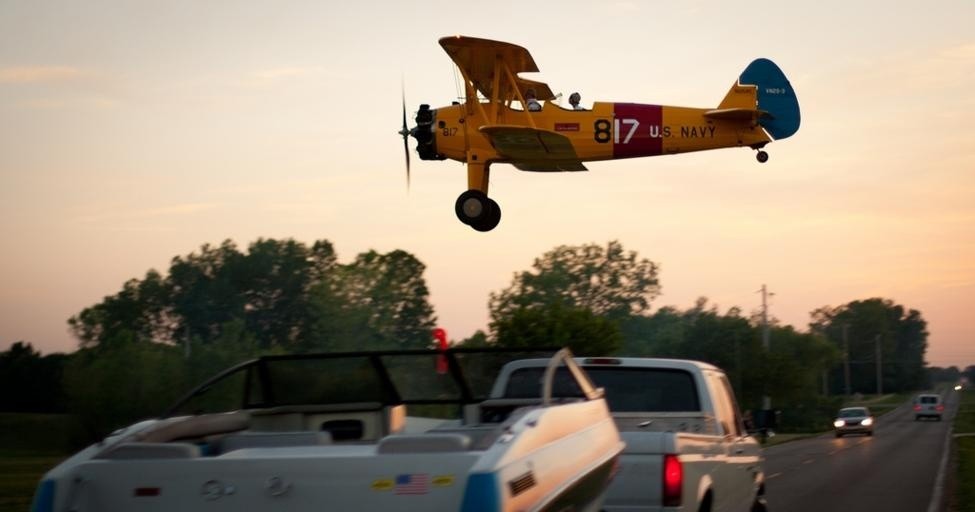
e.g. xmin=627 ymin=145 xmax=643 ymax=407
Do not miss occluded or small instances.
xmin=415 ymin=351 xmax=784 ymax=512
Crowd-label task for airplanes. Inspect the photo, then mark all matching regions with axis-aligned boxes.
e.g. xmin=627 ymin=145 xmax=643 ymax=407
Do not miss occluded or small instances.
xmin=396 ymin=31 xmax=802 ymax=232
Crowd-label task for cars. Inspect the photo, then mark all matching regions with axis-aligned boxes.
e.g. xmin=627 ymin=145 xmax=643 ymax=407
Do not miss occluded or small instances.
xmin=834 ymin=406 xmax=875 ymax=438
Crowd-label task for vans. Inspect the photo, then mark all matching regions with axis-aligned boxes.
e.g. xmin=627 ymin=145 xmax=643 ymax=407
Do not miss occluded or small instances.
xmin=913 ymin=393 xmax=944 ymax=422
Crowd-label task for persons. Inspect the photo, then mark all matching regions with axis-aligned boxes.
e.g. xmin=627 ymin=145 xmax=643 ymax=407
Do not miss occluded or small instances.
xmin=524 ymin=88 xmax=542 ymax=110
xmin=568 ymin=90 xmax=588 ymax=110
